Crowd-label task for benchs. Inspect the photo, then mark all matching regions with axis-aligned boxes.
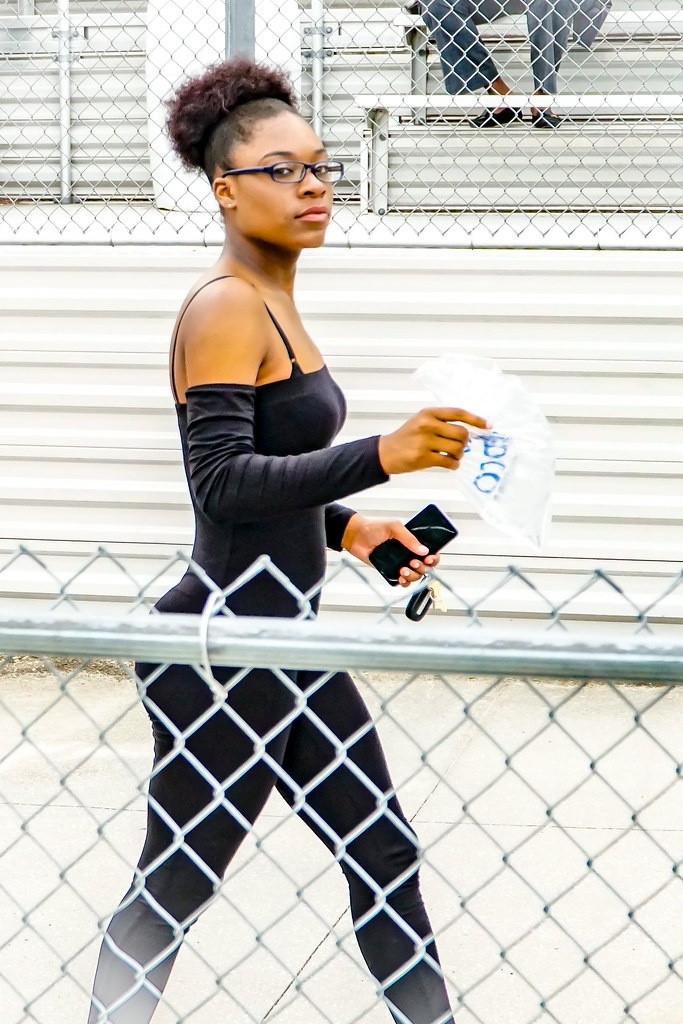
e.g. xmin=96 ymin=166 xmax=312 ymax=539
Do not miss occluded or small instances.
xmin=352 ymin=7 xmax=683 ymax=213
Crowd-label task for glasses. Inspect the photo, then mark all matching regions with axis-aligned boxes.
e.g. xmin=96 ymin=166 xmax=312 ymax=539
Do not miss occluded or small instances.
xmin=222 ymin=160 xmax=345 ymax=184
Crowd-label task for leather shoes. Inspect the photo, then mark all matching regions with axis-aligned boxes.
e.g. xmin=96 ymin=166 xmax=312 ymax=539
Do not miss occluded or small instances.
xmin=530 ymin=110 xmax=561 ymax=129
xmin=469 ymin=107 xmax=523 ymax=128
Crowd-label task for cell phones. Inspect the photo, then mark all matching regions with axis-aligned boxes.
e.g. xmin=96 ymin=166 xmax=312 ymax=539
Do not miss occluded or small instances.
xmin=368 ymin=503 xmax=459 ymax=587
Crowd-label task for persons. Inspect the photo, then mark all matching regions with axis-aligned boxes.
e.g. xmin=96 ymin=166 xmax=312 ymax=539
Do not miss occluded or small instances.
xmin=405 ymin=0 xmax=613 ymax=129
xmin=86 ymin=57 xmax=492 ymax=1024
